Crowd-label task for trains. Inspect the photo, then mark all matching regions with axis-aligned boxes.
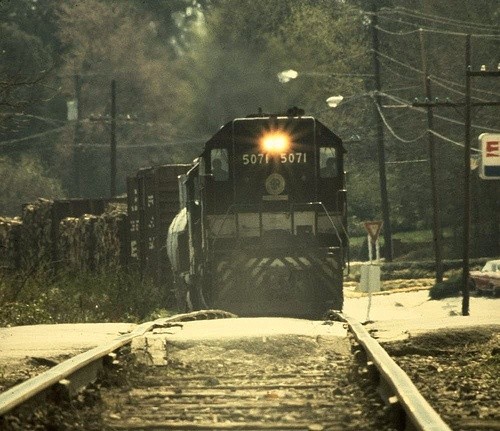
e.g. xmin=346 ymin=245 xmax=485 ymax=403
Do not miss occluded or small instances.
xmin=0 ymin=108 xmax=349 ymax=315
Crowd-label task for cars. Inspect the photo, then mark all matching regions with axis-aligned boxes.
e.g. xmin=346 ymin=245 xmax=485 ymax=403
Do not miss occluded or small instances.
xmin=468 ymin=259 xmax=499 ymax=296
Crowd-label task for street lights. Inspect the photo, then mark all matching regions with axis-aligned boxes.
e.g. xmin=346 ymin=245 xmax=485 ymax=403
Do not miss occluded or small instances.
xmin=275 ymin=66 xmax=393 ymax=267
